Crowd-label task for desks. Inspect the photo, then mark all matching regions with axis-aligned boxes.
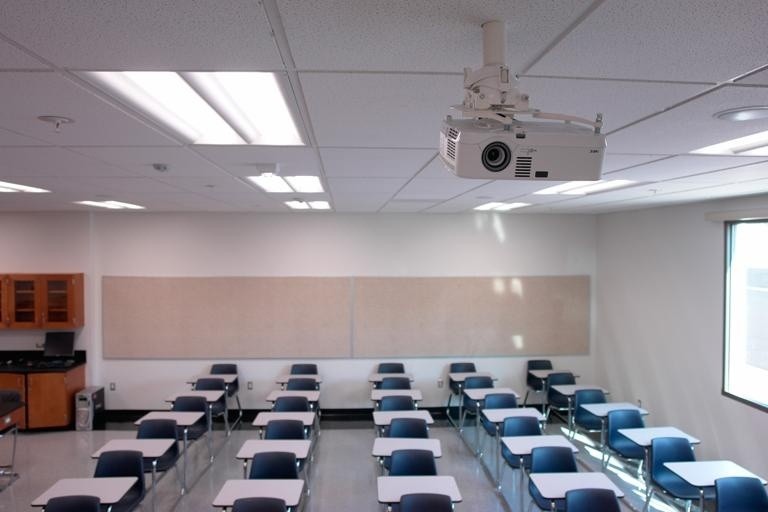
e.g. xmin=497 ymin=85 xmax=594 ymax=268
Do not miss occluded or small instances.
xmin=0 ymin=401 xmax=25 ymax=492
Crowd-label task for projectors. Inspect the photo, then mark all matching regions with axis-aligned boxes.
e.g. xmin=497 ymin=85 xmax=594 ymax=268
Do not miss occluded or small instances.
xmin=437 ymin=114 xmax=606 ymax=183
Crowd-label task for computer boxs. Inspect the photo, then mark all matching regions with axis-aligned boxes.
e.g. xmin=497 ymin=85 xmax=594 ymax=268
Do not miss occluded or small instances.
xmin=75 ymin=386 xmax=104 ymax=431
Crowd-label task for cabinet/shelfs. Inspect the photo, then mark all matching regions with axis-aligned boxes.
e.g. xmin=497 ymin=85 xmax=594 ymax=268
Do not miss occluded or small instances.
xmin=0 ymin=273 xmax=84 ymax=330
xmin=0 ymin=362 xmax=85 ymax=431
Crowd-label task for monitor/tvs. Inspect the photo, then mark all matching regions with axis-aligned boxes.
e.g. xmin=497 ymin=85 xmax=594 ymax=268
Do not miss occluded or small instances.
xmin=44 ymin=331 xmax=75 ymax=360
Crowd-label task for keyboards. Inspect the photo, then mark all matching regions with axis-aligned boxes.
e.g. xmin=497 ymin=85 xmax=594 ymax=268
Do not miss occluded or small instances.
xmin=39 ymin=360 xmax=68 ymax=368
